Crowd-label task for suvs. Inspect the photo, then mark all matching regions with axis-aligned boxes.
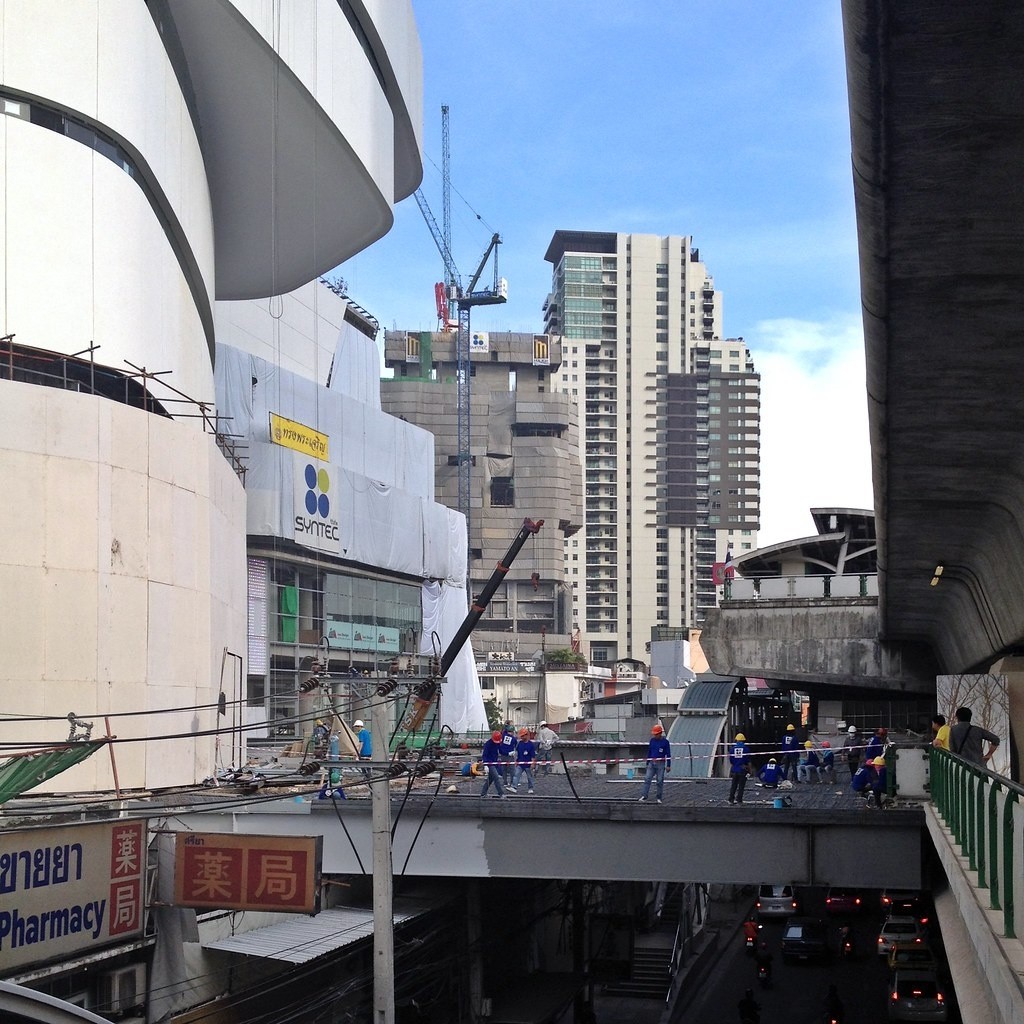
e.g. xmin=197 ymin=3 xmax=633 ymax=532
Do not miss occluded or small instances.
xmin=889 ymin=970 xmax=948 ymax=1024
xmin=891 ymin=942 xmax=938 ymax=979
xmin=779 ymin=916 xmax=831 ymax=967
xmin=878 ymin=914 xmax=924 ymax=958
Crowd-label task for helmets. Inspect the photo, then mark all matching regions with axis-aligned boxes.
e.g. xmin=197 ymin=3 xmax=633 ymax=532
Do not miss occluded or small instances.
xmin=822 ymin=741 xmax=830 ymax=747
xmin=492 ymin=731 xmax=502 ymax=743
xmin=506 ymin=725 xmax=515 ymax=731
xmin=735 ymin=733 xmax=746 ymax=741
xmin=866 ymin=759 xmax=873 ymax=765
xmin=870 ymin=756 xmax=885 ymax=765
xmin=878 ymin=728 xmax=887 ymax=734
xmin=804 ymin=741 xmax=812 ymax=747
xmin=539 ymin=721 xmax=546 ymax=726
xmin=847 ymin=725 xmax=856 ymax=732
xmin=787 ymin=724 xmax=795 ymax=730
xmin=353 ymin=720 xmax=363 ymax=726
xmin=769 ymin=758 xmax=777 ymax=763
xmin=519 ymin=729 xmax=527 ymax=736
xmin=651 ymin=725 xmax=662 ymax=734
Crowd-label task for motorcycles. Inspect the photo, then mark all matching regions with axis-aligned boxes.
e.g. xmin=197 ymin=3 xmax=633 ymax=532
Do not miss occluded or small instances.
xmin=754 ymin=960 xmax=772 ymax=982
xmin=740 ymin=924 xmax=763 ymax=952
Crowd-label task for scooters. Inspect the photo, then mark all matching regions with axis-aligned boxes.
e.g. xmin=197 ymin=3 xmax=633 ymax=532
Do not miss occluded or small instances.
xmin=738 ymin=1002 xmax=760 ymax=1024
xmin=822 ymin=1000 xmax=844 ymax=1024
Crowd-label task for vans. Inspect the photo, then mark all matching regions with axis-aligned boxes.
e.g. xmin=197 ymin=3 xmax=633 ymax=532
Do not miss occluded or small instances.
xmin=755 ymin=886 xmax=798 ymax=920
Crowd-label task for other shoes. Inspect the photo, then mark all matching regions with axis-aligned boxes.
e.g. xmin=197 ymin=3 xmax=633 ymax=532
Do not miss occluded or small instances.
xmin=500 ymin=794 xmax=510 ymax=801
xmin=527 ymin=789 xmax=533 ymax=794
xmin=656 ymin=799 xmax=662 ymax=803
xmin=727 ymin=800 xmax=733 ymax=806
xmin=481 ymin=794 xmax=492 ymax=798
xmin=738 ymin=802 xmax=747 ymax=805
xmin=638 ymin=796 xmax=646 ymax=801
xmin=506 ymin=787 xmax=517 ymax=794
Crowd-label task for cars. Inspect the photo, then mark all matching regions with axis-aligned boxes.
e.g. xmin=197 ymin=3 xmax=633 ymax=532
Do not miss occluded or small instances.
xmin=837 ymin=922 xmax=878 ymax=961
xmin=827 ymin=887 xmax=863 ymax=917
xmin=881 ymin=890 xmax=920 ymax=915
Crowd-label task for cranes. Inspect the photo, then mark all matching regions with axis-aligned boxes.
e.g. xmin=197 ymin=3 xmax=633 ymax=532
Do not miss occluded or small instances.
xmin=420 ymin=208 xmax=511 ymax=602
xmin=416 ymin=104 xmax=479 ymax=328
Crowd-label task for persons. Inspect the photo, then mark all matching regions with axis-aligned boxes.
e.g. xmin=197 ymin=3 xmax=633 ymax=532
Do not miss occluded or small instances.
xmin=316 ymin=719 xmax=373 ymax=780
xmin=481 ymin=721 xmax=559 ymax=801
xmin=950 ymin=708 xmax=1000 ymax=783
xmin=932 ymin=715 xmax=951 ymax=751
xmin=729 ymin=733 xmax=753 ymax=807
xmin=756 ymin=723 xmax=893 ymax=806
xmin=639 ymin=725 xmax=671 ymax=804
